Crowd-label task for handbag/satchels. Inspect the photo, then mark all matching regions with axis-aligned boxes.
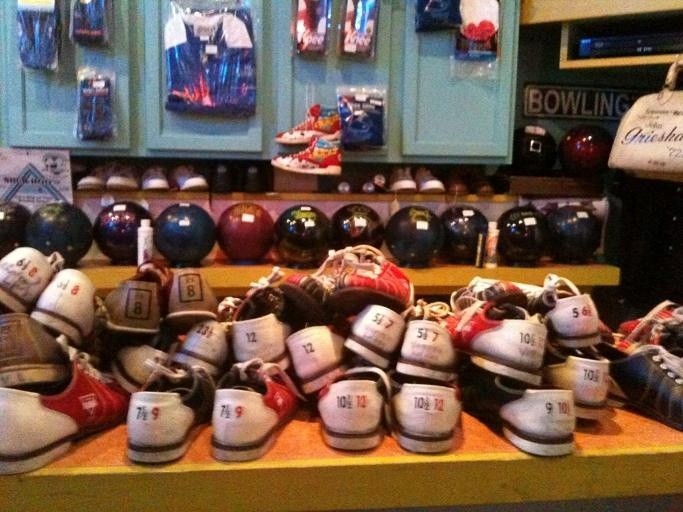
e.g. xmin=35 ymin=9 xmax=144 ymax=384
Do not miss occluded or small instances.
xmin=608 ymin=59 xmax=683 ymax=183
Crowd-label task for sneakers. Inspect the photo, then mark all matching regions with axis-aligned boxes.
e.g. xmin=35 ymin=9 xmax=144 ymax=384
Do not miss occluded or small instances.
xmin=76 ymin=160 xmax=266 ymax=193
xmin=271 ymin=135 xmax=342 ymax=176
xmin=273 ymin=104 xmax=342 ymax=144
xmin=390 ymin=167 xmax=493 ymax=196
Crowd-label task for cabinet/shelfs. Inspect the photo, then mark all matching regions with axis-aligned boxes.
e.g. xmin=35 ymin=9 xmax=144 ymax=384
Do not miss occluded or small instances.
xmin=0 ymin=260 xmax=683 ymax=510
